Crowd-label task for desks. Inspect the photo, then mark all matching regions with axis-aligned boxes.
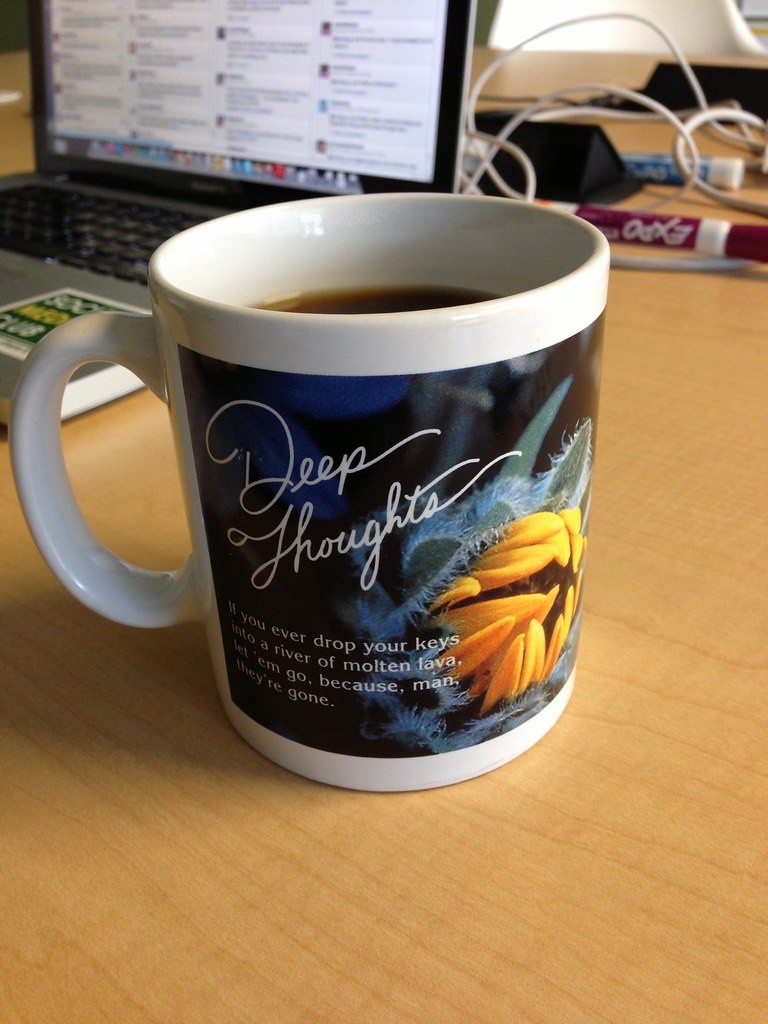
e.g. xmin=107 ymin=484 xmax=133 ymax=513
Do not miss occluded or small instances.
xmin=0 ymin=40 xmax=768 ymax=1024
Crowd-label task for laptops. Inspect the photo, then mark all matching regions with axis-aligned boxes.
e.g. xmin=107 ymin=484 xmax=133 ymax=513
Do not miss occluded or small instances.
xmin=0 ymin=0 xmax=475 ymax=431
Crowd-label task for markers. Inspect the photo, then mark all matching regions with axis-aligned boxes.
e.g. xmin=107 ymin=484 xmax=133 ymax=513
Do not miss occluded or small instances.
xmin=523 ymin=195 xmax=768 ymax=255
xmin=619 ymin=148 xmax=745 ymax=190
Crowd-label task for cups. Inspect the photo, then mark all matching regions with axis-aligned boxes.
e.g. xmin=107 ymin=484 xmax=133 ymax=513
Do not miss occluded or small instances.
xmin=8 ymin=193 xmax=611 ymax=793
